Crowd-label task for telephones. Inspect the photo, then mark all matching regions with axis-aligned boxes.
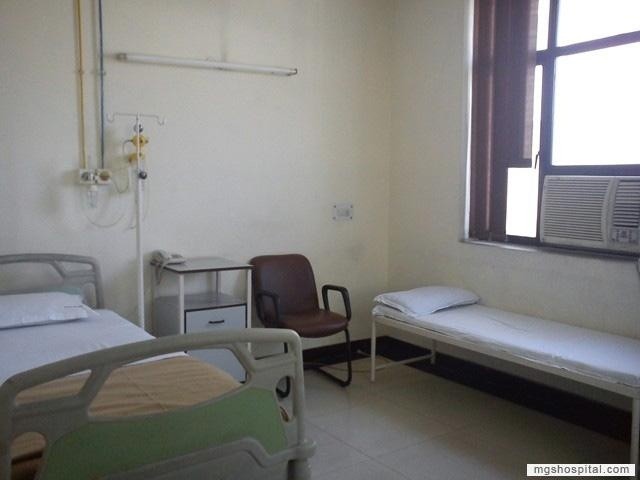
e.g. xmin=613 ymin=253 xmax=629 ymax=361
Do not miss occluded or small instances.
xmin=153 ymin=250 xmax=186 ymax=264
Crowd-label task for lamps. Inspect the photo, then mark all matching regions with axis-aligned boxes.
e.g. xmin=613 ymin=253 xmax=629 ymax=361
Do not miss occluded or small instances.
xmin=115 ymin=52 xmax=299 ymax=77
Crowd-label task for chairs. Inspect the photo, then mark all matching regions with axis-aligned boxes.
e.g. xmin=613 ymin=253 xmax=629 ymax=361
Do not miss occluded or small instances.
xmin=249 ymin=254 xmax=353 ymax=398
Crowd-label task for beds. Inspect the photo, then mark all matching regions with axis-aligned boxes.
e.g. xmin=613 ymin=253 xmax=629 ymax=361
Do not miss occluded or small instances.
xmin=370 ymin=305 xmax=640 ymax=480
xmin=0 ymin=252 xmax=317 ymax=480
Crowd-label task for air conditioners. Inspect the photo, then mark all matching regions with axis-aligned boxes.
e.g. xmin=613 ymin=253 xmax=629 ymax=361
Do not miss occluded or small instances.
xmin=537 ymin=175 xmax=640 ymax=258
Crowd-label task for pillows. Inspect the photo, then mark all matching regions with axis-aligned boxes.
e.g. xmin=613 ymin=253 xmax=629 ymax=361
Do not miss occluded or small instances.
xmin=372 ymin=285 xmax=481 ymax=318
xmin=0 ymin=291 xmax=89 ymax=330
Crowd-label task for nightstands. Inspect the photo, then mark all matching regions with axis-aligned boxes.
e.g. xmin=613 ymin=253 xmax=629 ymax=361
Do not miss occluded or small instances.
xmin=150 ymin=255 xmax=255 ymax=383
